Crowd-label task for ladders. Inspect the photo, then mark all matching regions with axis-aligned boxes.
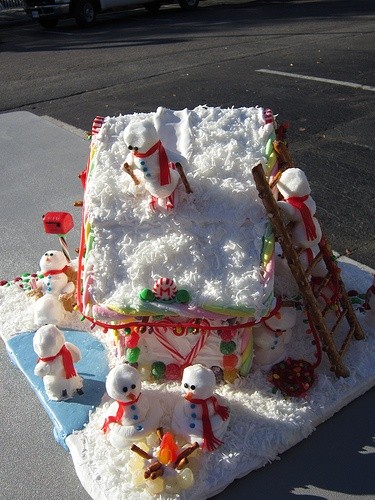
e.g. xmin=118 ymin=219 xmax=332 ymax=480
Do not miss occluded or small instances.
xmin=249 ymin=139 xmax=367 ymax=382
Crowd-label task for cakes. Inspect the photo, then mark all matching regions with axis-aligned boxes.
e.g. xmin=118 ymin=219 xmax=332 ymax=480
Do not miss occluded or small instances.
xmin=1 ymin=104 xmax=375 ymax=500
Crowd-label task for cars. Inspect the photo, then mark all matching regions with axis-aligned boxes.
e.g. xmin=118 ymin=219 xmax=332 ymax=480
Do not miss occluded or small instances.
xmin=20 ymin=0 xmax=206 ymax=31
